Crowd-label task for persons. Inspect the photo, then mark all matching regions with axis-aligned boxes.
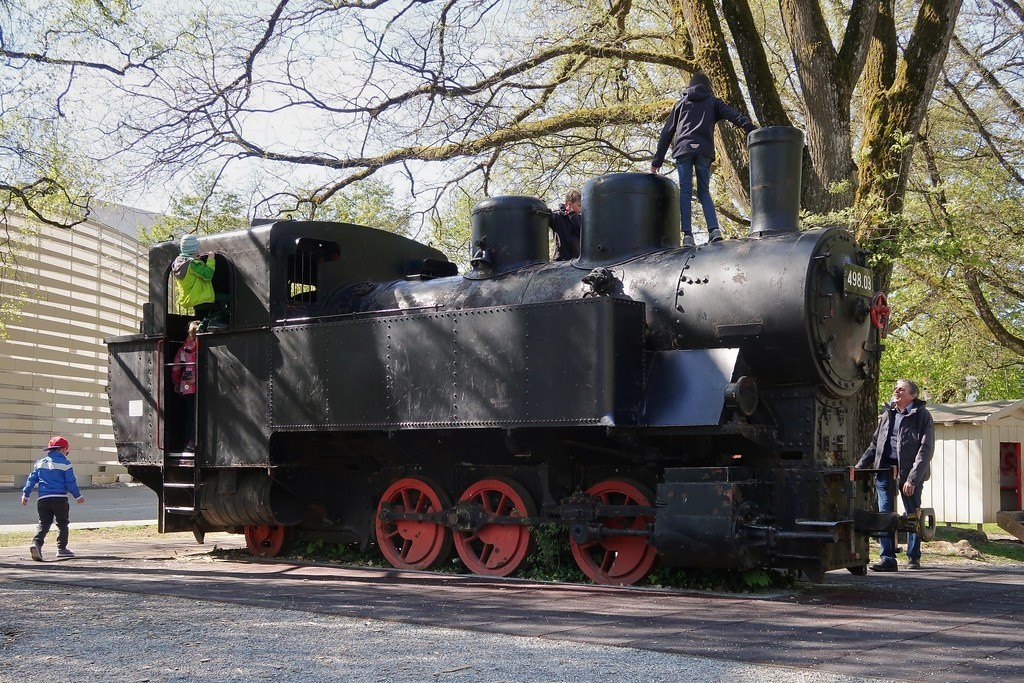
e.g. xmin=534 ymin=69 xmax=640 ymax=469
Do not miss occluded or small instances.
xmin=167 ymin=232 xmax=230 ymax=333
xmin=548 ymin=189 xmax=583 ymax=262
xmin=651 ymin=73 xmax=754 ymax=248
xmin=171 ymin=320 xmax=206 ymax=452
xmin=22 ymin=436 xmax=86 ymax=561
xmin=855 ymin=377 xmax=935 ymax=572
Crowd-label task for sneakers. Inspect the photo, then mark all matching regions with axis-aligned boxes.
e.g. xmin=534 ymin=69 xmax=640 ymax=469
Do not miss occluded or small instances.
xmin=708 ymin=229 xmax=724 ymax=243
xmin=196 ymin=317 xmax=227 ymax=333
xmin=683 ymin=236 xmax=695 ymax=247
xmin=56 ymin=550 xmax=74 ymax=557
xmin=30 ymin=542 xmax=44 ymax=561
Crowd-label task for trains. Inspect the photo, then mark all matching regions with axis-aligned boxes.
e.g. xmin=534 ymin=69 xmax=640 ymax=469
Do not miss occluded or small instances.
xmin=102 ymin=123 xmax=938 ymax=591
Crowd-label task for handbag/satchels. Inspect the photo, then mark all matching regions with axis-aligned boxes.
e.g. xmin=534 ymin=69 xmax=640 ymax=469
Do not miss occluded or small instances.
xmin=180 ymin=380 xmax=195 ymax=394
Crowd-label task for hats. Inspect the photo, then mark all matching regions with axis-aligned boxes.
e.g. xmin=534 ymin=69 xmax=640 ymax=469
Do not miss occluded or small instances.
xmin=42 ymin=436 xmax=69 ymax=451
xmin=180 ymin=234 xmax=200 ymax=257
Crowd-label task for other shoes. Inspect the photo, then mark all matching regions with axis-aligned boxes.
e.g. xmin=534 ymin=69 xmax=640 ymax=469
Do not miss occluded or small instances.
xmin=907 ymin=557 xmax=920 ymax=569
xmin=182 ymin=441 xmax=195 ymax=453
xmin=869 ymin=559 xmax=898 ymax=572
